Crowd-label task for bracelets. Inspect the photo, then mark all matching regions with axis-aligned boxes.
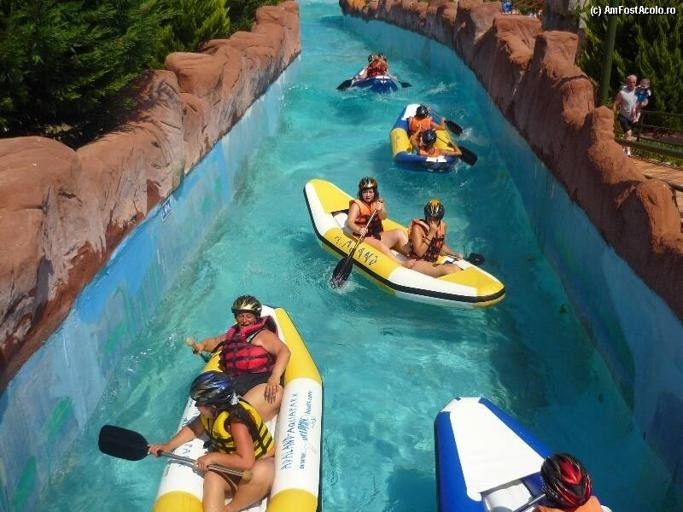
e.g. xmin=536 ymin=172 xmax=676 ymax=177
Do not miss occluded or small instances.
xmin=422 ymin=240 xmax=430 ymax=247
xmin=426 ymin=237 xmax=432 ymax=241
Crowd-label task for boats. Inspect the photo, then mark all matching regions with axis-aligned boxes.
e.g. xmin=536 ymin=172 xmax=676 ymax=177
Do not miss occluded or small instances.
xmin=342 ymin=65 xmax=403 ymax=92
xmin=350 ymin=75 xmax=401 ymax=96
xmin=390 ymin=103 xmax=473 ymax=174
xmin=390 ymin=103 xmax=457 ymax=172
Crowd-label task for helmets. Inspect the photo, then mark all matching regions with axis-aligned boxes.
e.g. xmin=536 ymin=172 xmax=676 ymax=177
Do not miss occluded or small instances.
xmin=189 ymin=371 xmax=236 ymax=404
xmin=539 ymin=452 xmax=591 ymax=510
xmin=358 ymin=176 xmax=377 ymax=190
xmin=421 ymin=128 xmax=436 ymax=145
xmin=416 ymin=105 xmax=428 ymax=117
xmin=423 ymin=200 xmax=445 ymax=218
xmin=230 ymin=295 xmax=263 ymax=319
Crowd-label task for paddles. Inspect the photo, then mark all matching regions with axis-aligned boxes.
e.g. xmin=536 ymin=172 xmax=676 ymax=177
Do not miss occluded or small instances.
xmin=336 ymin=69 xmax=363 ymax=92
xmin=98 ymin=422 xmax=254 ymax=485
xmin=330 ymin=197 xmax=384 ymax=292
xmin=384 ymin=72 xmax=414 ymax=90
xmin=440 ymin=252 xmax=485 ymax=267
xmin=415 ymin=122 xmax=478 ymax=166
xmin=430 ymin=107 xmax=464 ymax=138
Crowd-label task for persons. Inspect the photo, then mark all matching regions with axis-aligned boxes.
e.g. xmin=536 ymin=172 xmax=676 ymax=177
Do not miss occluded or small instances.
xmin=346 ymin=176 xmax=409 ymax=261
xmin=630 ymin=78 xmax=651 ymax=124
xmin=147 ymin=370 xmax=275 ymax=512
xmin=409 ymin=105 xmax=446 ymax=136
xmin=410 ymin=124 xmax=462 ymax=157
xmin=612 ymin=75 xmax=648 ymax=158
xmin=356 ymin=52 xmax=388 ymax=81
xmin=192 ymin=294 xmax=291 ymax=421
xmin=409 ymin=199 xmax=461 ymax=277
xmin=532 ymin=452 xmax=603 ymax=512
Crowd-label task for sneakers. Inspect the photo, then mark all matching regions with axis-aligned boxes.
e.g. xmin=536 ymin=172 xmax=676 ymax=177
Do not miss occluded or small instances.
xmin=623 ymin=148 xmax=632 ymax=157
